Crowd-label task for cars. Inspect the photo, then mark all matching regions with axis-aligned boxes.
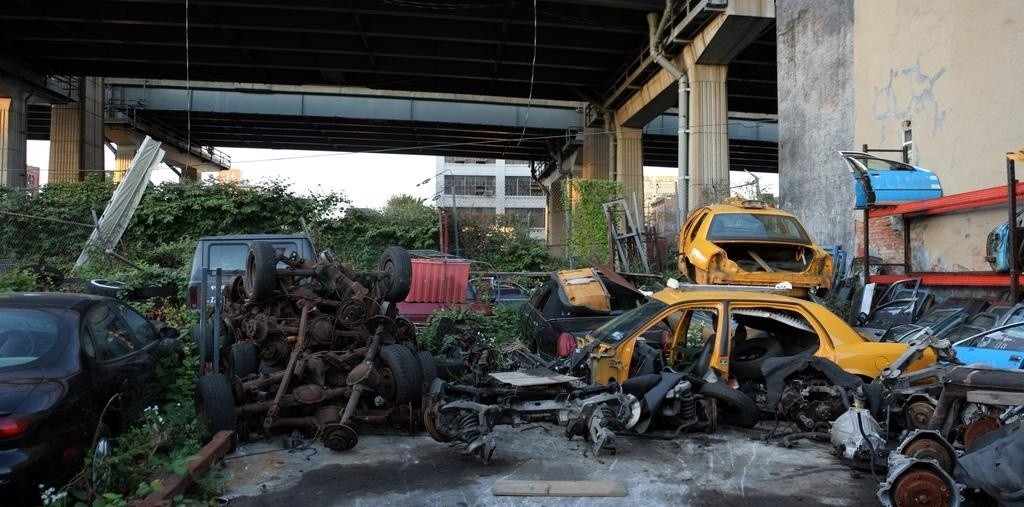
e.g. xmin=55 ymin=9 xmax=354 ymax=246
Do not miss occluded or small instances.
xmin=186 ymin=233 xmax=320 ymax=311
xmin=520 ymin=196 xmax=938 ymax=389
xmin=0 ymin=293 xmax=182 ymax=507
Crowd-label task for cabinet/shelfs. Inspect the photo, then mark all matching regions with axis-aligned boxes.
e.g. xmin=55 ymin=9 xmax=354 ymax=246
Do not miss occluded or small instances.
xmin=862 ymin=135 xmax=1023 ymax=311
